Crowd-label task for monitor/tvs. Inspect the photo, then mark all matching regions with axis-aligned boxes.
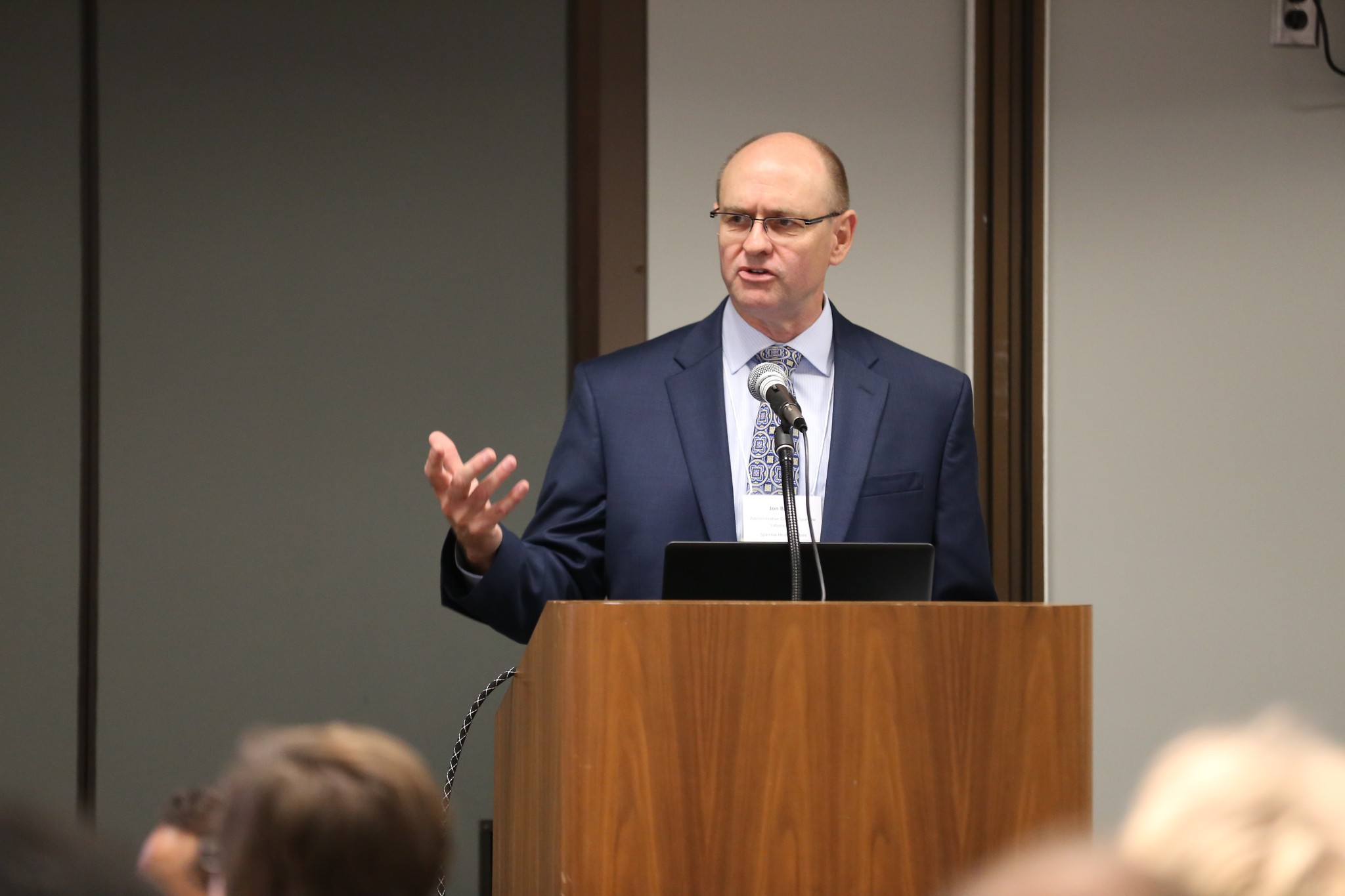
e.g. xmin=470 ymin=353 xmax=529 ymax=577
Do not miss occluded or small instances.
xmin=663 ymin=541 xmax=934 ymax=602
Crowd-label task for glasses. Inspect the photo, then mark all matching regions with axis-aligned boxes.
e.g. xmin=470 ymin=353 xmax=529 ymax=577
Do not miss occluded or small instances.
xmin=710 ymin=207 xmax=840 ymax=242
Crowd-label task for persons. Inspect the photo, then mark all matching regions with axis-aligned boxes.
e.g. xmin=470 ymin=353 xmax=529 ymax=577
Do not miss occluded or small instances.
xmin=423 ymin=131 xmax=993 ymax=647
xmin=0 ymin=722 xmax=442 ymax=896
xmin=970 ymin=716 xmax=1345 ymax=896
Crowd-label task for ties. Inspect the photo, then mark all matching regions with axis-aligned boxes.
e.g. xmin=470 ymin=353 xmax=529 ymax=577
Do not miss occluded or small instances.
xmin=747 ymin=345 xmax=803 ymax=497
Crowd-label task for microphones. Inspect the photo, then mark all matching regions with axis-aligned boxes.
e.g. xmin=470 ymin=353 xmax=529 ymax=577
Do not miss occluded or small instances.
xmin=747 ymin=362 xmax=808 ymax=433
